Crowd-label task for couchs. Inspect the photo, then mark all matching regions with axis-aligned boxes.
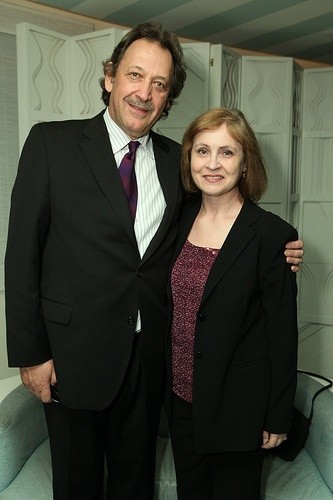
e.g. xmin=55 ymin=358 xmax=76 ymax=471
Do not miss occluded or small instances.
xmin=0 ymin=368 xmax=333 ymax=499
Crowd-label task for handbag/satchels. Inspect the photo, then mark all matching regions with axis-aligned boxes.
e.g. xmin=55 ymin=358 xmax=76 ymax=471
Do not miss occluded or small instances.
xmin=271 ymin=371 xmax=333 ymax=462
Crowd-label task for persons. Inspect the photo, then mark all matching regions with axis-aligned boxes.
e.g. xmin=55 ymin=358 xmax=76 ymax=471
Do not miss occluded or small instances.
xmin=4 ymin=22 xmax=304 ymax=500
xmin=165 ymin=107 xmax=297 ymax=500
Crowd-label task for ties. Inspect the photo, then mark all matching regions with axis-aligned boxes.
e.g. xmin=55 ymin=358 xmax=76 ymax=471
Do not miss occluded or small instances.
xmin=118 ymin=141 xmax=142 ymax=225
